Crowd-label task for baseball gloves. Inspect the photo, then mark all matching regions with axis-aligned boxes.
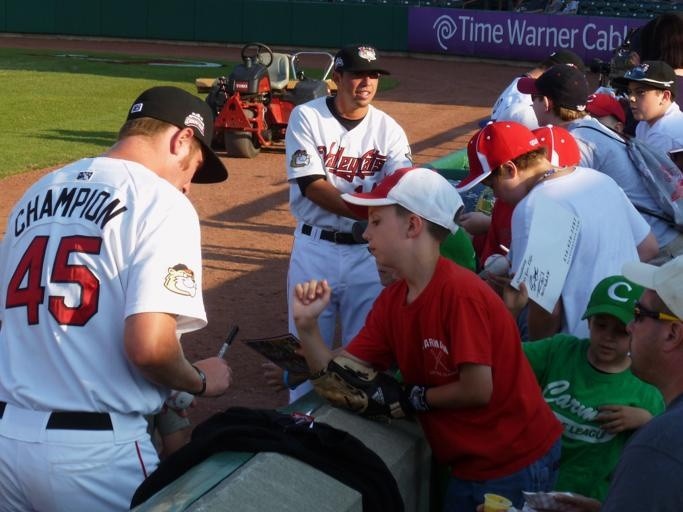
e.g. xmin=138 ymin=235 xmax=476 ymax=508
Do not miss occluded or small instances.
xmin=309 ymin=356 xmax=434 ymax=422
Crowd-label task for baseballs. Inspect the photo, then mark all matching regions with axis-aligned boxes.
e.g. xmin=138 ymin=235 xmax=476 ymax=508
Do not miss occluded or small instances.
xmin=166 ymin=387 xmax=194 ymax=410
xmin=485 ymin=254 xmax=510 ymax=276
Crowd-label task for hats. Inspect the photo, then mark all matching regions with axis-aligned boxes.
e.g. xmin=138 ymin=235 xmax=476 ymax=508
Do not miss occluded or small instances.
xmin=340 ymin=166 xmax=465 ymax=237
xmin=334 ymin=46 xmax=392 ymax=75
xmin=456 ymin=50 xmax=676 ymax=193
xmin=581 ymin=255 xmax=682 ymax=326
xmin=127 ymin=86 xmax=228 ymax=183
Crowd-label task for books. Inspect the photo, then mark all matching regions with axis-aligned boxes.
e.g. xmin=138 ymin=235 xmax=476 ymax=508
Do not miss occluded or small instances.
xmin=241 ymin=332 xmax=311 ymax=376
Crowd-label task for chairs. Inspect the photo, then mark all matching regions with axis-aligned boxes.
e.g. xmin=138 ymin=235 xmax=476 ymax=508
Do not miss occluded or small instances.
xmin=253 ymin=52 xmax=290 ymax=90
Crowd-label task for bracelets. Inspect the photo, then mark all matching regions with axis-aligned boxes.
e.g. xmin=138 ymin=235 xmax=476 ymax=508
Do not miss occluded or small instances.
xmin=188 ymin=363 xmax=206 ymax=395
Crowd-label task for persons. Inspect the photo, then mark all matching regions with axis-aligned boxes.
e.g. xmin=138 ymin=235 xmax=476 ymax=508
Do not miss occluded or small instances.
xmin=0 ymin=85 xmax=234 ymax=512
xmin=292 ymin=244 xmax=405 ymax=387
xmin=513 ymin=0 xmax=578 ymax=14
xmin=291 ymin=168 xmax=565 ymax=512
xmin=449 ymin=0 xmax=474 ymax=9
xmin=475 ymin=255 xmax=682 ymax=512
xmin=462 ymin=42 xmax=683 ymax=339
xmin=285 ymin=43 xmax=413 ymax=403
xmin=500 ymin=272 xmax=668 ymax=503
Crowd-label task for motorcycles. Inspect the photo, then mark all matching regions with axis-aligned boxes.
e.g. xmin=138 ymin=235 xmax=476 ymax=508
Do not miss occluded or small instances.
xmin=205 ymin=40 xmax=332 ymax=159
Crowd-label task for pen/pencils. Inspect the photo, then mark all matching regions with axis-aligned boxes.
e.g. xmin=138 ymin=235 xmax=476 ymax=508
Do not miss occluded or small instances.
xmin=218 ymin=325 xmax=239 ymax=358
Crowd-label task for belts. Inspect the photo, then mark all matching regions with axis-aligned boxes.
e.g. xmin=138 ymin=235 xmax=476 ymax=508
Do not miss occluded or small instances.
xmin=1 ymin=401 xmax=113 ymax=430
xmin=302 ymin=224 xmax=368 ymax=245
